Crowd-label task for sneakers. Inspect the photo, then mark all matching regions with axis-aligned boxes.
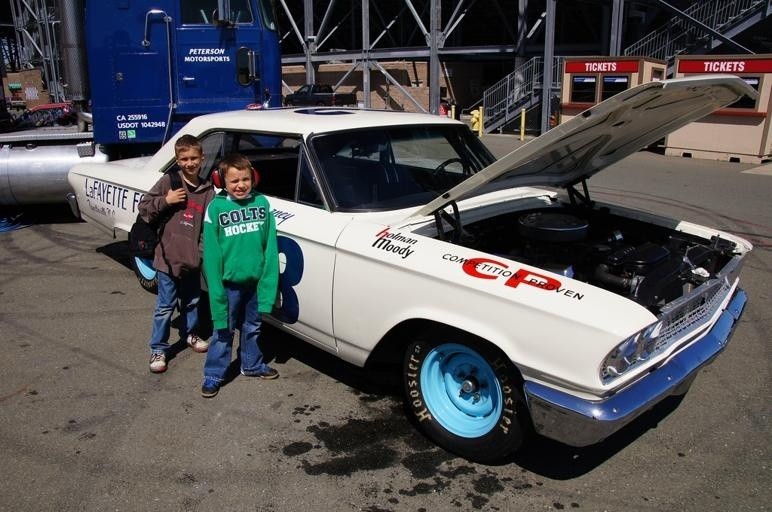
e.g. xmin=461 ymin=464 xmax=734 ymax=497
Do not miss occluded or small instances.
xmin=241 ymin=366 xmax=278 ymax=379
xmin=180 ymin=334 xmax=208 ymax=352
xmin=149 ymin=353 xmax=166 ymax=373
xmin=202 ymin=380 xmax=220 ymax=397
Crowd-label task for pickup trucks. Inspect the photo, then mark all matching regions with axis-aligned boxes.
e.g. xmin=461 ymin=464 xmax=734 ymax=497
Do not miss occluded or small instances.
xmin=284 ymin=83 xmax=357 ymax=107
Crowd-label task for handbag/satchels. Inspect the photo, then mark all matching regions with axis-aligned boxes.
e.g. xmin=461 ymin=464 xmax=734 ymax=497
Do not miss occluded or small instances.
xmin=129 ymin=214 xmax=157 ymax=260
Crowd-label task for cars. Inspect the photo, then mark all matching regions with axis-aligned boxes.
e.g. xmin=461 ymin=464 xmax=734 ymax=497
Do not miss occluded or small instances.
xmin=63 ymin=70 xmax=762 ymax=464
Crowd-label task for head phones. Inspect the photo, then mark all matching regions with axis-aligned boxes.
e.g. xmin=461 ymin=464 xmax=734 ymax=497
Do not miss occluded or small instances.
xmin=211 ymin=167 xmax=261 ymax=188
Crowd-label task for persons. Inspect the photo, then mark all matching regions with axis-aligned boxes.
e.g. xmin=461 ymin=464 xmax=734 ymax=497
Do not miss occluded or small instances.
xmin=200 ymin=152 xmax=282 ymax=397
xmin=138 ymin=134 xmax=218 ymax=374
